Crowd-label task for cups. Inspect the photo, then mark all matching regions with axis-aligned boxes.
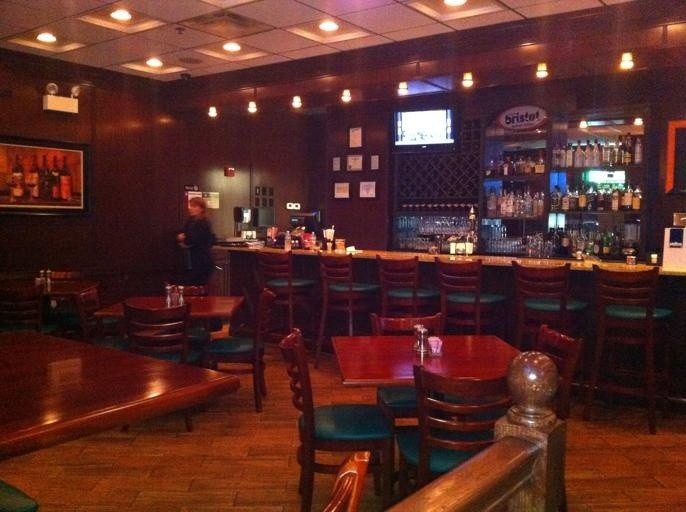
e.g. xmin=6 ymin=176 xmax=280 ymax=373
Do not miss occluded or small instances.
xmin=240 ymin=230 xmax=265 ymax=250
xmin=673 ymin=211 xmax=686 ymax=227
xmin=392 ymin=215 xmax=522 ymax=255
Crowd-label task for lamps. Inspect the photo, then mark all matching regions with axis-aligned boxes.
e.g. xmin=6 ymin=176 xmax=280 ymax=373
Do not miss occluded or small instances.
xmin=40 ymin=82 xmax=82 ymax=115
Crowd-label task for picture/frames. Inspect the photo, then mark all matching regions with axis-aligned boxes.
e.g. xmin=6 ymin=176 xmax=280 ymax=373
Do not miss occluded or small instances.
xmin=0 ymin=134 xmax=93 ymax=221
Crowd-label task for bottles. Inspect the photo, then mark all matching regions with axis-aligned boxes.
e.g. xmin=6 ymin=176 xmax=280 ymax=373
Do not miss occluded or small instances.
xmin=33 ymin=267 xmax=53 ymax=293
xmin=162 ymin=282 xmax=185 ymax=308
xmin=2 ymin=152 xmax=73 ymax=203
xmin=266 ymin=226 xmax=346 ymax=253
xmin=521 ymin=224 xmax=638 ymax=265
xmin=409 ymin=321 xmax=432 ymax=358
xmin=551 ymin=181 xmax=642 ymax=211
xmin=484 ymin=149 xmax=544 ymax=217
xmin=548 ymin=133 xmax=644 ymax=168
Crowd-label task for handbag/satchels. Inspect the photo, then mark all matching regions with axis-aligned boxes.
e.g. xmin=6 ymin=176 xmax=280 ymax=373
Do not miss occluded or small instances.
xmin=171 ymin=243 xmax=210 ymax=277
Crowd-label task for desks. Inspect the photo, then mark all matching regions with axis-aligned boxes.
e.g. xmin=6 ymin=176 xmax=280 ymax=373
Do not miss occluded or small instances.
xmin=326 ymin=330 xmax=525 ymax=412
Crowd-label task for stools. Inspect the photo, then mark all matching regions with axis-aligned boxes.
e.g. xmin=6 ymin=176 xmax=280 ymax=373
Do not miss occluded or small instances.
xmin=251 ymin=248 xmax=313 ymax=349
xmin=505 ymin=258 xmax=578 ymax=418
xmin=432 ymin=255 xmax=503 ymax=342
xmin=313 ymin=248 xmax=378 ymax=349
xmin=580 ymin=262 xmax=674 ymax=435
xmin=372 ymin=253 xmax=441 ymax=329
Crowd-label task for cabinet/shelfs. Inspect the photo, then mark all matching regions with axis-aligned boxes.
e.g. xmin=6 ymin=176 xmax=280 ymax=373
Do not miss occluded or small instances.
xmin=478 ymin=125 xmax=648 ymax=251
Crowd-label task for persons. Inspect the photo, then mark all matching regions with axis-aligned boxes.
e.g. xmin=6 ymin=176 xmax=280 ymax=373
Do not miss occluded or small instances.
xmin=176 ymin=198 xmax=217 ymax=285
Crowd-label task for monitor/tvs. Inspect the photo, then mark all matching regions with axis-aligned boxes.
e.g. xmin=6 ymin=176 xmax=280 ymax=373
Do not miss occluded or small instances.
xmin=391 ymin=104 xmax=457 ymax=155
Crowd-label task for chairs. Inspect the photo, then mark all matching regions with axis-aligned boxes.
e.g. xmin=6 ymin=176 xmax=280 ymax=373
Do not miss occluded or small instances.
xmin=275 ymin=325 xmax=401 ymax=510
xmin=0 ymin=245 xmax=275 ymax=466
xmin=366 ymin=309 xmax=451 ymax=497
xmin=527 ymin=322 xmax=587 ymax=509
xmin=394 ymin=356 xmax=518 ymax=512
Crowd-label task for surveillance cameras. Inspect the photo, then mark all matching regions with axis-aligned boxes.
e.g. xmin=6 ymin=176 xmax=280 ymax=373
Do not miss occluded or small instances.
xmin=180 ymin=72 xmax=192 ymax=80
